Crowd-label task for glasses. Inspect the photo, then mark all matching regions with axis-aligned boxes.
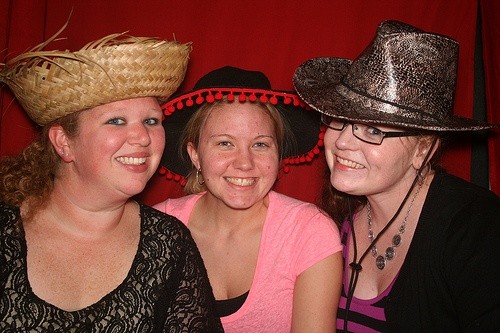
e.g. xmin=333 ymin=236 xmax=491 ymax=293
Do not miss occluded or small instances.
xmin=321 ymin=112 xmax=434 ymax=145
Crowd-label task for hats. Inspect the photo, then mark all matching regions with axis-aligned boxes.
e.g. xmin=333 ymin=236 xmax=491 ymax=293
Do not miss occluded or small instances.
xmin=155 ymin=65 xmax=328 ymax=186
xmin=0 ymin=12 xmax=193 ymax=126
xmin=292 ymin=19 xmax=495 ymax=132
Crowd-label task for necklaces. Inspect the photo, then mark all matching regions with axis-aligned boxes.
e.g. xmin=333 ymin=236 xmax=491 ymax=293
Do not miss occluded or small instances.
xmin=367 ymin=164 xmax=432 ymax=270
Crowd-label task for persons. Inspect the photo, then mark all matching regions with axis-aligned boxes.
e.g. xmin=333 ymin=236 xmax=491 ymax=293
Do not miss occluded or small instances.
xmin=0 ymin=33 xmax=225 ymax=333
xmin=293 ymin=20 xmax=500 ymax=333
xmin=151 ymin=66 xmax=343 ymax=333
